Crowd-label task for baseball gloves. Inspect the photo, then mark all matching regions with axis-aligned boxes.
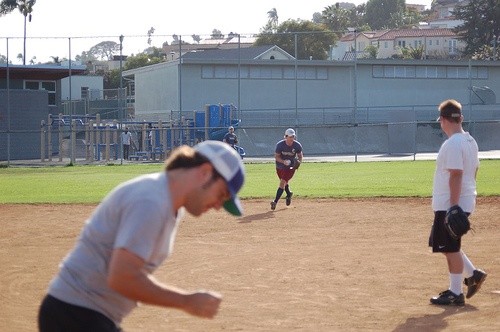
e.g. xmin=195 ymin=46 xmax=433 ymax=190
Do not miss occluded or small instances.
xmin=289 ymin=157 xmax=300 ymax=171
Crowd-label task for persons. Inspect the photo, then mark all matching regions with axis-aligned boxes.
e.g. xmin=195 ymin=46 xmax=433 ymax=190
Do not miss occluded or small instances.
xmin=37 ymin=139 xmax=247 ymax=332
xmin=428 ymin=99 xmax=489 ymax=306
xmin=223 ymin=126 xmax=239 ymax=149
xmin=120 ymin=127 xmax=132 ymax=160
xmin=145 ymin=122 xmax=152 ymax=147
xmin=271 ymin=128 xmax=303 ymax=210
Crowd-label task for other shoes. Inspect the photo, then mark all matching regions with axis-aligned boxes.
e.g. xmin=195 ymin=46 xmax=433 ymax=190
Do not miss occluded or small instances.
xmin=271 ymin=201 xmax=276 ymax=210
xmin=286 ymin=191 xmax=293 ymax=206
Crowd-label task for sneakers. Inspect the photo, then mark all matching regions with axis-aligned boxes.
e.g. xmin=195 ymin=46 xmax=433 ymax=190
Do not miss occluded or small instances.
xmin=430 ymin=290 xmax=464 ymax=306
xmin=463 ymin=269 xmax=487 ymax=299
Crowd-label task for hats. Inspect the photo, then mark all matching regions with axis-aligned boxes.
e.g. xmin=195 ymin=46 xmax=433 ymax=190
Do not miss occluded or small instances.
xmin=436 ymin=111 xmax=462 ymax=122
xmin=285 ymin=129 xmax=295 ymax=136
xmin=194 ymin=140 xmax=245 ymax=217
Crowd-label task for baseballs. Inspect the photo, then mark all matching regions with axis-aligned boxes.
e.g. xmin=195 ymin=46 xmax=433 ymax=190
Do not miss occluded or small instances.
xmin=286 ymin=160 xmax=291 ymax=166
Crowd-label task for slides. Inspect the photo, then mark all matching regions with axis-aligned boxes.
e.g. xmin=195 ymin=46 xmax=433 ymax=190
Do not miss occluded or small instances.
xmin=208 ymin=117 xmax=246 ymax=160
